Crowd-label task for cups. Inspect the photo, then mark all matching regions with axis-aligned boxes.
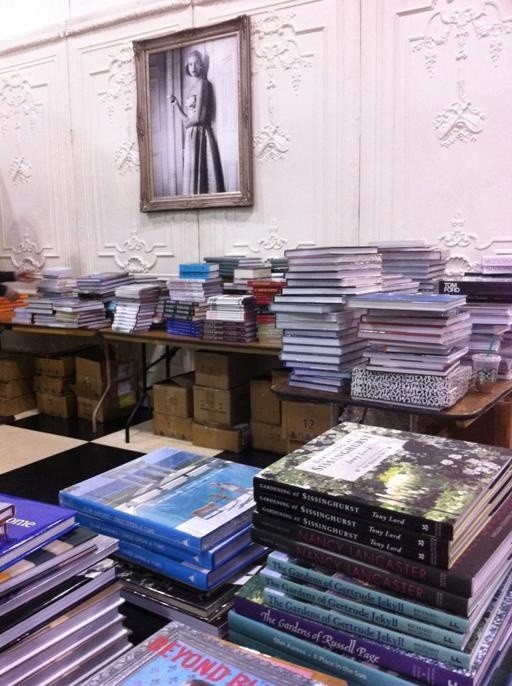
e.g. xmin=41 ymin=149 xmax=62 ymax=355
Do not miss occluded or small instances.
xmin=471 ymin=353 xmax=503 ymax=395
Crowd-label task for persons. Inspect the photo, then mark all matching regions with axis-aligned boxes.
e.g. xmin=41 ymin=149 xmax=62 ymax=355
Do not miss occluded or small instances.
xmin=168 ymin=49 xmax=221 ymax=195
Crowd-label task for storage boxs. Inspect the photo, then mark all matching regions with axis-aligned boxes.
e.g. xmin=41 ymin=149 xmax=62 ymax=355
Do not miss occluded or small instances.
xmin=190 ymin=353 xmax=339 ymax=454
xmin=1 ymin=347 xmax=35 ymax=416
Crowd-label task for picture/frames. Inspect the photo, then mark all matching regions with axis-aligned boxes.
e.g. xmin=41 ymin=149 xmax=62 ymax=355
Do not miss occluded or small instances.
xmin=132 ymin=16 xmax=253 ymax=212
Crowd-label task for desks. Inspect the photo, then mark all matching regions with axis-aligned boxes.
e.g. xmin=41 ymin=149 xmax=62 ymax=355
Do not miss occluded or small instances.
xmin=11 ymin=325 xmax=510 ymax=442
